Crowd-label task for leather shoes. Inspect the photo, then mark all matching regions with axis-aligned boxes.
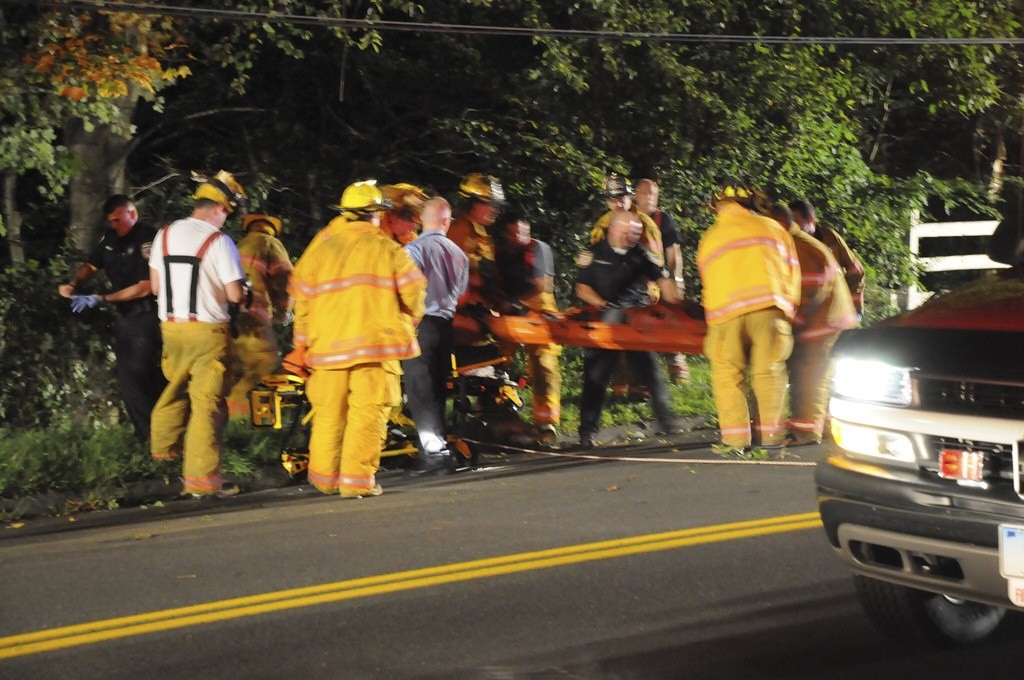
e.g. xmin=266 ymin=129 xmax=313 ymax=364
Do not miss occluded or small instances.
xmin=405 ymin=458 xmax=452 ymax=478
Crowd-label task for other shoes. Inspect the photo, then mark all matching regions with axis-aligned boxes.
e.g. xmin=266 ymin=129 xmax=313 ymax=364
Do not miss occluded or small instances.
xmin=785 ymin=432 xmax=817 ymax=446
xmin=579 ymin=432 xmax=595 ymax=449
xmin=540 ymin=423 xmax=558 ymax=444
xmin=666 ymin=427 xmax=685 ymax=435
xmin=710 ymin=442 xmax=782 ymax=457
xmin=188 ymin=482 xmax=242 ymax=496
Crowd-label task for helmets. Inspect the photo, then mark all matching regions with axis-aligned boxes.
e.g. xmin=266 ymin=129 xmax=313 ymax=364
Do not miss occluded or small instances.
xmin=328 ymin=181 xmax=393 ymax=216
xmin=242 ymin=214 xmax=282 ymax=236
xmin=599 ymin=172 xmax=634 ymax=197
xmin=192 ymin=170 xmax=245 ymax=212
xmin=456 ymin=172 xmax=510 ymax=206
xmin=379 ymin=183 xmax=432 ymax=224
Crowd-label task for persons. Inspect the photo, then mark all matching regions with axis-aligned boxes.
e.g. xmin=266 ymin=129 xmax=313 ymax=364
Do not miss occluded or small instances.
xmin=571 ymin=211 xmax=682 ymax=448
xmin=762 ymin=202 xmax=858 ymax=447
xmin=60 ymin=195 xmax=169 ymax=439
xmin=378 ymin=169 xmax=565 ymax=474
xmin=589 ymin=172 xmax=690 ymax=405
xmin=788 ymin=200 xmax=866 ymax=317
xmin=282 ymin=180 xmax=429 ymax=498
xmin=224 ymin=214 xmax=294 ymax=428
xmin=148 ymin=167 xmax=251 ymax=500
xmin=697 ymin=177 xmax=803 ymax=459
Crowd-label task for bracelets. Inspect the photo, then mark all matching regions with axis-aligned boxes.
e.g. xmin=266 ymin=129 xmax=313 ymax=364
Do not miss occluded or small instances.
xmin=68 ymin=283 xmax=75 ymax=288
xmin=102 ymin=295 xmax=107 ymax=301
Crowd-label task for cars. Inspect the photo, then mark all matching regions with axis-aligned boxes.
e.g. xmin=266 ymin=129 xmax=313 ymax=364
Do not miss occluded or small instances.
xmin=818 ymin=272 xmax=1024 ymax=652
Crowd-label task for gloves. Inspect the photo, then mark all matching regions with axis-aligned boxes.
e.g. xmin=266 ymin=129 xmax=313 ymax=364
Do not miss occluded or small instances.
xmin=69 ymin=295 xmax=98 ymax=313
